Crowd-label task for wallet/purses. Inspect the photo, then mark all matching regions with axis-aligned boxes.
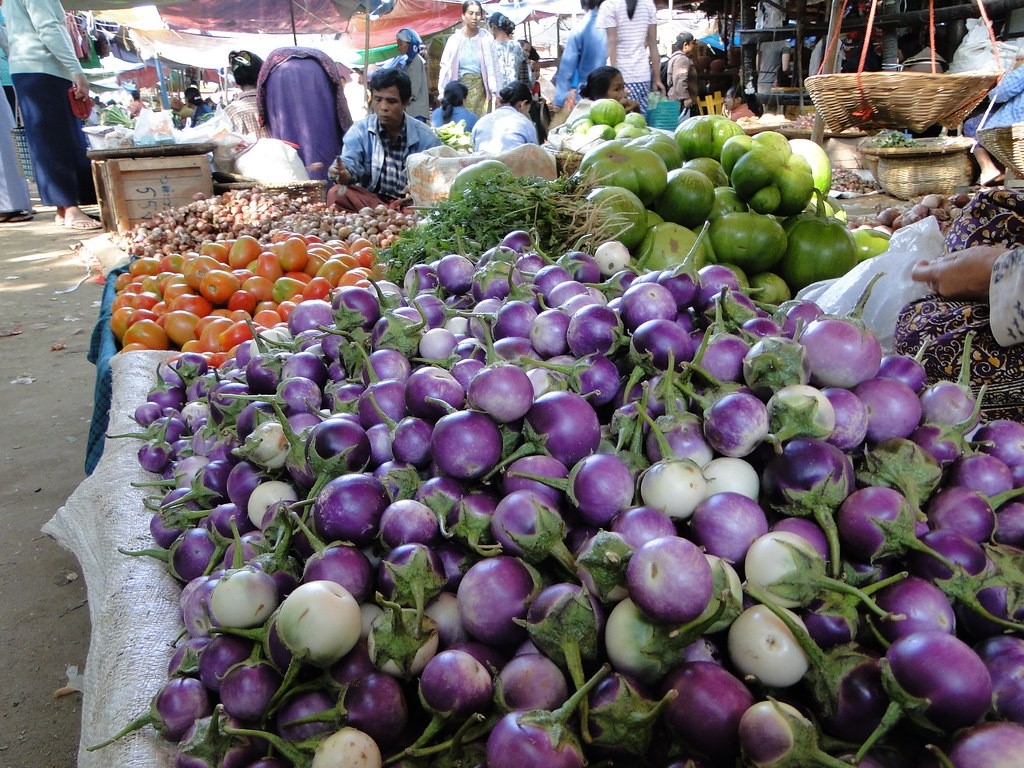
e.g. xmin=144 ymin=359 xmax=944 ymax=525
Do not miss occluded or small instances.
xmin=68 ymin=86 xmax=94 ymax=119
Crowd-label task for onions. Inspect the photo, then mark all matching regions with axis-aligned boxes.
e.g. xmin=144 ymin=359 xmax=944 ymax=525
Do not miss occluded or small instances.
xmin=847 ymin=193 xmax=971 ymax=237
xmin=118 ymin=187 xmax=419 ymax=260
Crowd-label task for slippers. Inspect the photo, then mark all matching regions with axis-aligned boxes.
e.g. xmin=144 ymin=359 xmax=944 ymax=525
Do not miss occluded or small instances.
xmin=0 ymin=213 xmax=34 ymax=223
xmin=56 ymin=217 xmax=103 ymax=229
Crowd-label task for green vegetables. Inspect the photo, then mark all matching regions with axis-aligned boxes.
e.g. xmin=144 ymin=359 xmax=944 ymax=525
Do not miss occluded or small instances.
xmin=873 ymin=130 xmax=929 ymax=147
xmin=370 ymin=167 xmax=619 ymax=276
xmin=427 ymin=119 xmax=474 ymax=152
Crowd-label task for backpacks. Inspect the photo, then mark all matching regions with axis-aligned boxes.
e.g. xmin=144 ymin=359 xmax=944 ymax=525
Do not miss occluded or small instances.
xmin=659 ymin=53 xmax=685 ymax=96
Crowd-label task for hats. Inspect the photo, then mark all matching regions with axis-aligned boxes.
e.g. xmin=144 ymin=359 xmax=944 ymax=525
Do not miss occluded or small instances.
xmin=185 ymin=87 xmax=204 ymax=106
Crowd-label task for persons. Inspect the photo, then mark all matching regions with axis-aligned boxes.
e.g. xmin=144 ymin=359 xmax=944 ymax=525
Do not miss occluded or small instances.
xmin=472 ymin=81 xmax=539 ymax=152
xmin=660 ymin=41 xmax=742 ymax=101
xmin=595 ymin=0 xmax=666 ymax=115
xmin=257 ymin=46 xmax=353 ymax=181
xmin=964 ymin=61 xmax=1024 ymax=185
xmin=756 ymin=25 xmax=951 ymax=93
xmin=724 ymin=87 xmax=755 ymax=121
xmin=77 ymin=87 xmax=237 ymax=148
xmin=566 ymin=66 xmax=641 ymax=131
xmin=2 ymin=0 xmax=103 ymax=230
xmin=667 ymin=30 xmax=698 ymax=113
xmin=432 ymin=81 xmax=477 ymax=133
xmin=553 ymin=0 xmax=609 ymax=112
xmin=438 ymin=0 xmax=551 ymax=145
xmin=327 ymin=69 xmax=440 ymax=215
xmin=344 ymin=28 xmax=431 ymax=125
xmin=891 ymin=190 xmax=1024 ymax=424
xmin=0 ymin=0 xmax=37 ymax=223
xmin=222 ymin=50 xmax=271 ymax=142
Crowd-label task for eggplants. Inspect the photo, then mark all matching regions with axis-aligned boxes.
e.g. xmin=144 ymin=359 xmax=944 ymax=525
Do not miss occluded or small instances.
xmin=117 ymin=230 xmax=1024 ymax=767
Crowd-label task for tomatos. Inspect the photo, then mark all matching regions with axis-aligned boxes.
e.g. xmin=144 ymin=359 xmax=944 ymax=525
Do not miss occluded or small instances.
xmin=108 ymin=231 xmax=388 ymax=364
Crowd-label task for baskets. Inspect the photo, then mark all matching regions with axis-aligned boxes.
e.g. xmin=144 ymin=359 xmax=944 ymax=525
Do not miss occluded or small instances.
xmin=977 ymin=124 xmax=1024 ymax=179
xmin=859 ymin=136 xmax=979 ymax=202
xmin=803 ymin=71 xmax=1003 ymax=135
